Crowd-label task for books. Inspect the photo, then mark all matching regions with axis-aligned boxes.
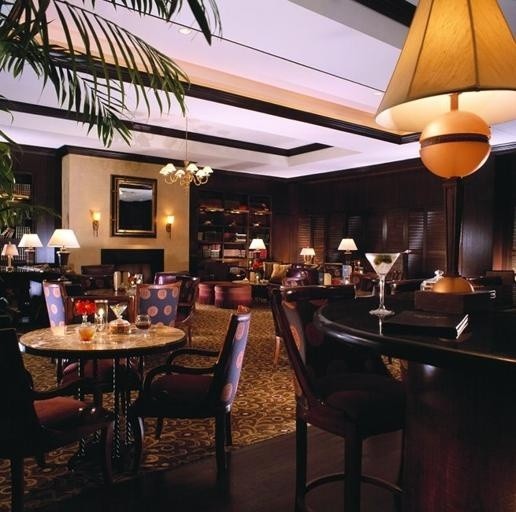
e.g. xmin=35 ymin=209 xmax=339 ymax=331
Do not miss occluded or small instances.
xmin=381 ymin=310 xmax=469 ymax=340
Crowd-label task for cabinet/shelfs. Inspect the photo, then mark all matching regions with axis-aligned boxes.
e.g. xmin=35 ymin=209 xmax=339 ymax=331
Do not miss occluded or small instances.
xmin=190 ymin=189 xmax=272 ymax=280
xmin=273 ymin=178 xmax=456 ymax=280
xmin=0 ymin=171 xmax=60 ymax=264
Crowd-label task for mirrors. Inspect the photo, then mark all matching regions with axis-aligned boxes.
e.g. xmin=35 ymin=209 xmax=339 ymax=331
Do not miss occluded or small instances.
xmin=112 ymin=176 xmax=157 ymax=237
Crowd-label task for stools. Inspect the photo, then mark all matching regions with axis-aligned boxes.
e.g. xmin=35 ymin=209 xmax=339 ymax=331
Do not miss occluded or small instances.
xmin=214 ymin=284 xmax=252 ymax=306
xmin=198 ymin=282 xmax=232 ymax=304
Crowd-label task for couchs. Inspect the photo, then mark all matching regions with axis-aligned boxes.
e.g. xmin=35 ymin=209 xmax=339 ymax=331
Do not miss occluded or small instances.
xmin=261 ymin=263 xmax=320 ymax=306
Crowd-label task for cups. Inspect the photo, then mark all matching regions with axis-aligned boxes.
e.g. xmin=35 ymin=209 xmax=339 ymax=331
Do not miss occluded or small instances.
xmin=364 ymin=252 xmax=402 ymax=316
xmin=77 ymin=325 xmax=97 ymax=344
xmin=134 ymin=314 xmax=152 ymax=338
xmin=50 ymin=324 xmax=67 ymax=339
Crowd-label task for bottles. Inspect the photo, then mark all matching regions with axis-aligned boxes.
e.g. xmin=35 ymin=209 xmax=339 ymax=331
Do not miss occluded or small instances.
xmin=123 ymin=271 xmax=130 ymax=283
xmin=249 ymin=267 xmax=256 ymax=283
xmin=114 ymin=272 xmax=122 ymax=291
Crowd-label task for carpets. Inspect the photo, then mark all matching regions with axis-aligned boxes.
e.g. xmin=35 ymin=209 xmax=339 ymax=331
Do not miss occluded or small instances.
xmin=0 ymin=305 xmax=404 ymax=510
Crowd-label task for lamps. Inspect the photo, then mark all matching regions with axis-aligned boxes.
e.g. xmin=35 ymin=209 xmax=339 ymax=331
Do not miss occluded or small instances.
xmin=160 ymin=116 xmax=213 ymax=188
xmin=338 ymin=238 xmax=357 ymax=263
xmin=166 ymin=216 xmax=174 ymax=232
xmin=300 ymin=248 xmax=315 ymax=266
xmin=93 ymin=212 xmax=101 ymax=231
xmin=47 ymin=229 xmax=81 ymax=272
xmin=18 ymin=234 xmax=43 ymax=266
xmin=1 ymin=244 xmax=19 ymax=272
xmin=249 ymin=238 xmax=266 ymax=267
xmin=373 ymin=0 xmax=515 ymax=295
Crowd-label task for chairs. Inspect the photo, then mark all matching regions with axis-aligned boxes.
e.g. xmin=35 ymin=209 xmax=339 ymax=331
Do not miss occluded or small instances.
xmin=0 ymin=328 xmax=115 ymax=512
xmin=154 ymin=272 xmax=199 ymax=348
xmin=43 ymin=278 xmax=82 ymax=364
xmin=125 ymin=280 xmax=182 ymax=382
xmin=81 ymin=265 xmax=117 ymax=286
xmin=129 ymin=306 xmax=252 ymax=512
xmin=271 ymin=289 xmax=406 ymax=512
